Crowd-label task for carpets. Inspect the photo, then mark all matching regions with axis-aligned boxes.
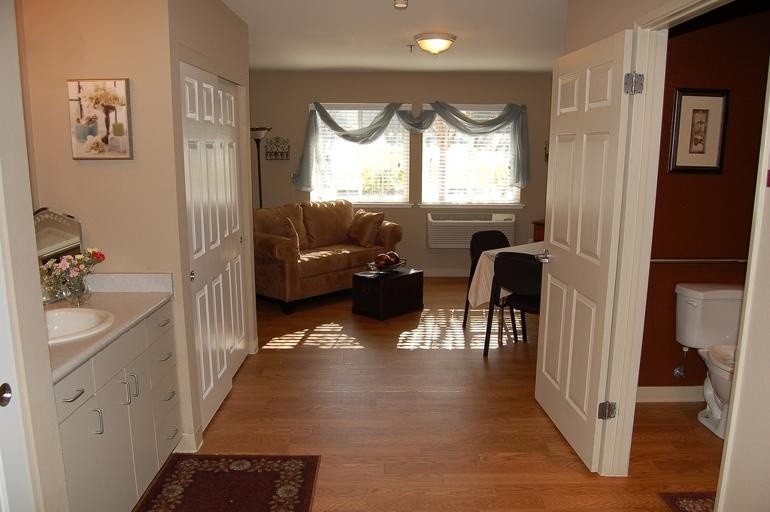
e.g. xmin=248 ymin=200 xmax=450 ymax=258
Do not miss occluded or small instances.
xmin=658 ymin=491 xmax=715 ymax=512
xmin=132 ymin=452 xmax=321 ymax=512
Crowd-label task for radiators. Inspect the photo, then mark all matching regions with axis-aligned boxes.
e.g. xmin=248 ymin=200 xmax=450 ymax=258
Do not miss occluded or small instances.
xmin=427 ymin=214 xmax=516 ymax=248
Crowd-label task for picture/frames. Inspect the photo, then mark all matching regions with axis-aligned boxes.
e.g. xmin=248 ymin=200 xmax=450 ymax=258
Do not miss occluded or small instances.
xmin=66 ymin=78 xmax=133 ymax=161
xmin=668 ymin=87 xmax=730 ymax=174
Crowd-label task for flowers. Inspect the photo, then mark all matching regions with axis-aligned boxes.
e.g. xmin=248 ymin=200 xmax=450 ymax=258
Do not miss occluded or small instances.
xmin=39 ymin=247 xmax=105 ymax=294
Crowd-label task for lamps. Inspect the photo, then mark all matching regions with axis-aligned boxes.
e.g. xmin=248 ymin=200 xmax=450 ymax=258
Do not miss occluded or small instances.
xmin=415 ymin=32 xmax=456 ymax=56
xmin=250 ymin=127 xmax=272 ymax=208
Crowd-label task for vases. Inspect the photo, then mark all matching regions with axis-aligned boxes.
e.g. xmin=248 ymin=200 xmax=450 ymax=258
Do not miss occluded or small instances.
xmin=63 ymin=282 xmax=93 ymax=304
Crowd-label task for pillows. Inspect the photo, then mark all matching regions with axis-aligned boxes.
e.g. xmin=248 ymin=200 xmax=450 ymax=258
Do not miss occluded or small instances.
xmin=254 ymin=216 xmax=300 ymax=257
xmin=252 ymin=203 xmax=309 ymax=248
xmin=303 ymin=199 xmax=352 ymax=247
xmin=350 ymin=208 xmax=383 ymax=248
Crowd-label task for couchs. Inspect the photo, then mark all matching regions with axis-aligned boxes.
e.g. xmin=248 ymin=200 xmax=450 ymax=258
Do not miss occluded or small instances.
xmin=253 ymin=219 xmax=402 ymax=314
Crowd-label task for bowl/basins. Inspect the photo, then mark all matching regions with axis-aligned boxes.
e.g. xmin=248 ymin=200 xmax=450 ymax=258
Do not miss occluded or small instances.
xmin=367 ymin=259 xmax=406 ymax=271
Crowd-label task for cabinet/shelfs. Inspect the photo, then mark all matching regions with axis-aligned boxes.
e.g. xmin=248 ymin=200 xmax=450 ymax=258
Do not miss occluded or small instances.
xmin=55 ymin=294 xmax=194 ymax=512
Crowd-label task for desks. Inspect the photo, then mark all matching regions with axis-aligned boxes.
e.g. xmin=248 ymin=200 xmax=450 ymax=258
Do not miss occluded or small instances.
xmin=467 ymin=241 xmax=544 ymax=343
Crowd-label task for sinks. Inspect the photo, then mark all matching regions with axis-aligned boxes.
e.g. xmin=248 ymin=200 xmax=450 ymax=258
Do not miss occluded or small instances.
xmin=44 ymin=307 xmax=115 ymax=344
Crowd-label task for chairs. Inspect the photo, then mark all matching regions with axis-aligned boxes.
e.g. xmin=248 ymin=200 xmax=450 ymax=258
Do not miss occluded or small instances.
xmin=464 ymin=231 xmax=518 ymax=344
xmin=481 ymin=252 xmax=542 ymax=357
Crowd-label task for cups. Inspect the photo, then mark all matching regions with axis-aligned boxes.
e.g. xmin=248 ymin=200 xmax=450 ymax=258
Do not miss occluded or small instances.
xmin=112 ymin=122 xmax=125 ymax=137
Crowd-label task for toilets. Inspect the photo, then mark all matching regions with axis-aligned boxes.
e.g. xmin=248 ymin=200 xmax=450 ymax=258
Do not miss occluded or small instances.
xmin=673 ymin=282 xmax=744 ymax=441
